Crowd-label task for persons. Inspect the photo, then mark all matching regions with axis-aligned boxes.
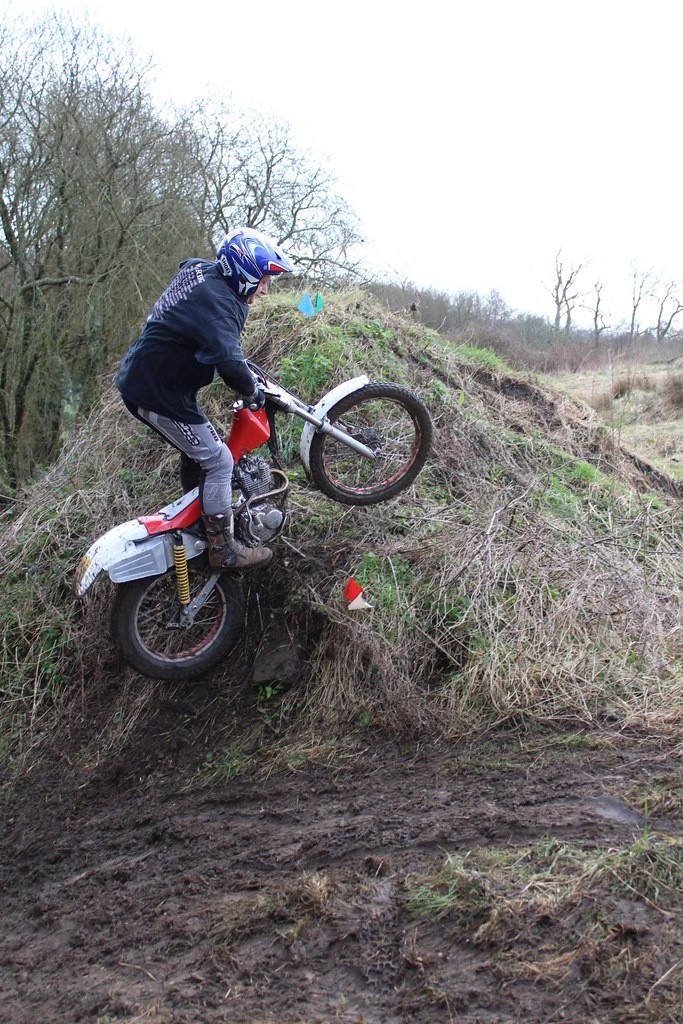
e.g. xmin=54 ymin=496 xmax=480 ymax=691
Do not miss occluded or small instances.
xmin=116 ymin=227 xmax=295 ymax=571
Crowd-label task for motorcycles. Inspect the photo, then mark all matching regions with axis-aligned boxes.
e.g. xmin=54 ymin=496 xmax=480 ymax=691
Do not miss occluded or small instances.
xmin=68 ymin=364 xmax=437 ymax=685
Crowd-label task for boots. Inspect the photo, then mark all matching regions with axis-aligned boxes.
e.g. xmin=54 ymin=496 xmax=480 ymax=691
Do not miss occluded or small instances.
xmin=200 ymin=508 xmax=272 ymax=573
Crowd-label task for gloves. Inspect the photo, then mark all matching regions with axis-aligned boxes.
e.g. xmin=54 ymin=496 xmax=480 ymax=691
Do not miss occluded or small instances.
xmin=244 ymin=389 xmax=265 ymax=411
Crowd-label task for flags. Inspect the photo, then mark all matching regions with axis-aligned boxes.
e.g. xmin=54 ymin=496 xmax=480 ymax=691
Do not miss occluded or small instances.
xmin=343 ymin=577 xmax=374 ymax=611
xmin=297 ymin=290 xmax=324 ymax=317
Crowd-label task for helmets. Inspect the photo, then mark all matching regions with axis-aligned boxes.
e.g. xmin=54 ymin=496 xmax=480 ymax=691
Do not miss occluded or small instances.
xmin=214 ymin=226 xmax=292 ymax=302
